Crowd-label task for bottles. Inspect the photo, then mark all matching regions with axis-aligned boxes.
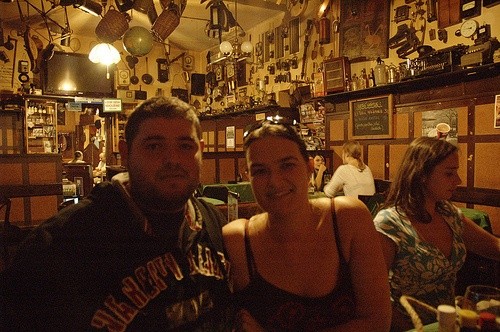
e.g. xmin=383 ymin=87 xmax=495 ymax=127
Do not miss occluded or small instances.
xmin=351 ymin=55 xmax=407 ymax=91
xmin=437 ymin=304 xmax=500 ymax=332
xmin=26 ymin=102 xmax=55 ymax=126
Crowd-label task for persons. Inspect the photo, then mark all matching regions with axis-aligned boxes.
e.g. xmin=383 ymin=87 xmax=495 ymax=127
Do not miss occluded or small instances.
xmin=323 ymin=140 xmax=375 ymax=199
xmin=309 ymin=152 xmax=331 ymax=192
xmin=0 ymin=96 xmax=241 ymax=332
xmin=95 ymin=152 xmax=106 ymax=173
xmin=373 ymin=136 xmax=500 ymax=332
xmin=68 ymin=150 xmax=85 ymax=164
xmin=222 ymin=115 xmax=392 ymax=332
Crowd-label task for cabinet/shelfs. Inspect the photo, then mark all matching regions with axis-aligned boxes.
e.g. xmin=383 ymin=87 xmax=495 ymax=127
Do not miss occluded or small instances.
xmin=24 ymin=97 xmax=58 ymax=153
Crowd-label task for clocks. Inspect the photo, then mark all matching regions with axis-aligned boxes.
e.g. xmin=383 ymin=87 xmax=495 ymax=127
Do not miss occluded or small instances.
xmin=460 ymin=19 xmax=479 ymax=38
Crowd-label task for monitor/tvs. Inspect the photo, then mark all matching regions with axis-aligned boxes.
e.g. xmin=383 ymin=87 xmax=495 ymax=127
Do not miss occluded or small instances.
xmin=40 ymin=51 xmax=114 ymax=99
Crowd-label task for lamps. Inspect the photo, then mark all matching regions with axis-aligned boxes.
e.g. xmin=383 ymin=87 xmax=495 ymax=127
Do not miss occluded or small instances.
xmin=236 ymin=41 xmax=253 ymax=62
xmin=207 ymin=40 xmax=233 ymax=66
xmin=88 ymin=0 xmax=121 ymax=66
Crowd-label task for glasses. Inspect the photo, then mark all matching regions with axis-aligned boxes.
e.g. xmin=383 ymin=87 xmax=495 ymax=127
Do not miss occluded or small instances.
xmin=242 ymin=115 xmax=303 ymax=142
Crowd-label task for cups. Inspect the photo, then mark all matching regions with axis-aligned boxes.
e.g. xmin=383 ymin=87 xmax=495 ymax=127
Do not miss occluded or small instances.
xmin=462 ymin=285 xmax=500 ymax=314
xmin=428 ymin=123 xmax=450 ymax=140
